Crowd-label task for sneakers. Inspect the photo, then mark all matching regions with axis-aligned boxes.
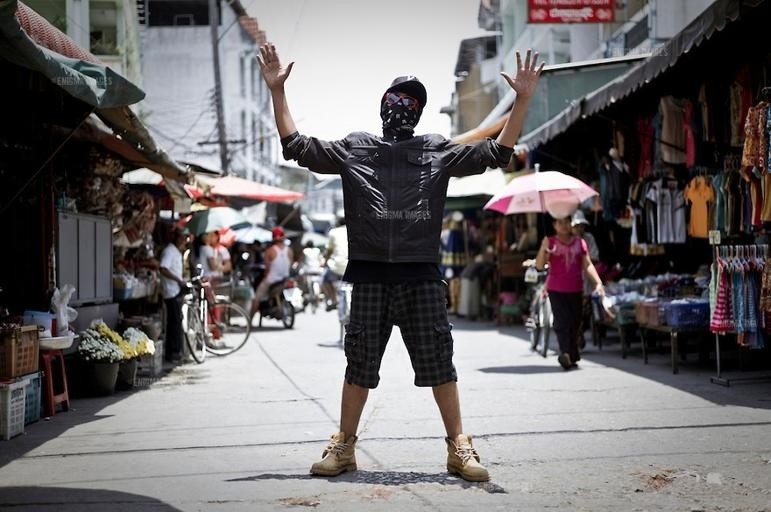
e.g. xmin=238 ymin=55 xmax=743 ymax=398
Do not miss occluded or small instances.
xmin=558 ymin=353 xmax=578 ymax=371
xmin=444 ymin=432 xmax=490 ymax=483
xmin=308 ymin=429 xmax=358 ymax=477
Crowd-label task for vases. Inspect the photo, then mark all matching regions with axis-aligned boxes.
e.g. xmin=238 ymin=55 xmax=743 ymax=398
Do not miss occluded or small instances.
xmin=118 ymin=356 xmax=137 ymax=391
xmin=81 ymin=358 xmax=119 ymax=398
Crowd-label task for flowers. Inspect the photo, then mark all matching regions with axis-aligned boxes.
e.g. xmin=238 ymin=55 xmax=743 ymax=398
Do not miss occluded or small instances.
xmin=77 ymin=317 xmax=132 ymax=364
xmin=118 ymin=327 xmax=155 ymax=363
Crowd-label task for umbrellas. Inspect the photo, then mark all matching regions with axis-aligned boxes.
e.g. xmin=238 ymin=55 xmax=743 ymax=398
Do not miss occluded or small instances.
xmin=485 ymin=171 xmax=600 ymax=253
xmin=185 ymin=207 xmax=248 ymax=235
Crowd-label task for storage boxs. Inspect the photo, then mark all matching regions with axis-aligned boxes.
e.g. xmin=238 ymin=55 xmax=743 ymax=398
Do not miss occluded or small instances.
xmin=0 ymin=379 xmax=29 ymax=444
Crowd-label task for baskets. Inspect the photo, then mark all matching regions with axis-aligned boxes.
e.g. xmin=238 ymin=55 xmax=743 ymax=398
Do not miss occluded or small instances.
xmin=0 ymin=378 xmax=31 ymax=441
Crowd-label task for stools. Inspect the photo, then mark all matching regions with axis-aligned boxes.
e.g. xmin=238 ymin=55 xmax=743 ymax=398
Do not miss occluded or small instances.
xmin=37 ymin=349 xmax=70 ymax=418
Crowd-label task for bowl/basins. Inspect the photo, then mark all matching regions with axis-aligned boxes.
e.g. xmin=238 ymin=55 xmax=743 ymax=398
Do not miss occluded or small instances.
xmin=40 ymin=334 xmax=79 ymax=349
xmin=23 ymin=311 xmax=52 ymax=337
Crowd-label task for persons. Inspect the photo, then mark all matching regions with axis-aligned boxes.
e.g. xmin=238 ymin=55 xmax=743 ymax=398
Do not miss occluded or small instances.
xmin=570 ymin=209 xmax=600 ymax=349
xmin=255 ymin=41 xmax=546 ymax=481
xmin=161 ymin=219 xmax=350 ymax=364
xmin=536 ymin=213 xmax=606 ymax=371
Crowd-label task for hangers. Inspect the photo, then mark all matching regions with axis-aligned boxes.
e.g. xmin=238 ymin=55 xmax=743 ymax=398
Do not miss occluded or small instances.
xmin=754 ymin=86 xmax=771 ymax=106
xmin=637 ymin=156 xmax=743 ymax=182
xmin=713 ymin=242 xmax=770 ymax=274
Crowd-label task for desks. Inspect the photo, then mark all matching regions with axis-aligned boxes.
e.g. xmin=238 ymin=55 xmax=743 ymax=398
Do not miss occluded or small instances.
xmin=489 ymin=258 xmax=527 ymax=306
xmin=636 ymin=322 xmax=688 ymax=375
xmin=594 ymin=316 xmax=638 ymax=361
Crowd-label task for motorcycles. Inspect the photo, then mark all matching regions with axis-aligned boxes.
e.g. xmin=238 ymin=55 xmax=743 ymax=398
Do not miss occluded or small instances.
xmin=240 ymin=251 xmax=297 ymax=333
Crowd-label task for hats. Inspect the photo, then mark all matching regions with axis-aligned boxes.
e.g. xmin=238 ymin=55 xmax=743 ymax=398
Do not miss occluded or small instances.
xmin=571 ymin=207 xmax=591 ymax=228
xmin=271 ymin=225 xmax=286 ymax=242
xmin=170 ymin=226 xmax=192 ymax=239
xmin=385 ymin=75 xmax=427 ymax=109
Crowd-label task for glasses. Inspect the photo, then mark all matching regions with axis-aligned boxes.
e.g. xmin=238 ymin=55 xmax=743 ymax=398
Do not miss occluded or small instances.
xmin=385 ymin=92 xmax=421 ymax=109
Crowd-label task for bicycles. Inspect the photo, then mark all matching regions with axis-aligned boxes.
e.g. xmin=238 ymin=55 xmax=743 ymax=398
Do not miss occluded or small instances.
xmin=523 ymin=256 xmax=553 ymax=360
xmin=318 ymin=260 xmax=351 ymax=345
xmin=291 ymin=259 xmax=322 ymax=315
xmin=176 ymin=266 xmax=252 ymax=370
